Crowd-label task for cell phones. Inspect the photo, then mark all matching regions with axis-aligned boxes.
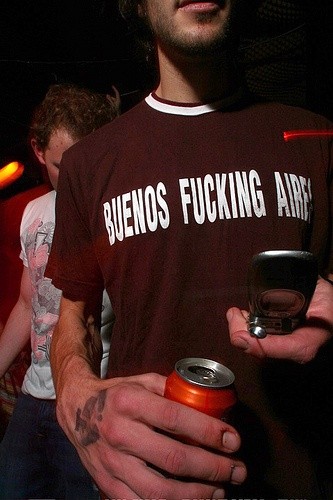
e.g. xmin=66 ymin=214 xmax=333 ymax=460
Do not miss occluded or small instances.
xmin=248 ymin=250 xmax=317 ymax=339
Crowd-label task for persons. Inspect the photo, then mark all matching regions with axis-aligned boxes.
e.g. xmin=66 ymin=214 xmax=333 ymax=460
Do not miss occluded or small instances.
xmin=0 ymin=85 xmax=120 ymax=500
xmin=44 ymin=0 xmax=333 ymax=500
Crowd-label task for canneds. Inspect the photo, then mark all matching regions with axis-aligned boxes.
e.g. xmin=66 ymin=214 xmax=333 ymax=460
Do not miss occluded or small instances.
xmin=161 ymin=357 xmax=237 ymax=448
